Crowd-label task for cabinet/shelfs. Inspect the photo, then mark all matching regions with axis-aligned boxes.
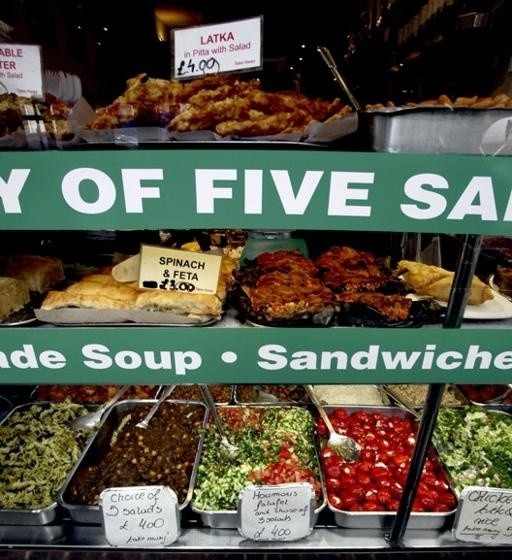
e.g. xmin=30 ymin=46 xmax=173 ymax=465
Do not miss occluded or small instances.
xmin=1 ymin=146 xmax=512 ymax=558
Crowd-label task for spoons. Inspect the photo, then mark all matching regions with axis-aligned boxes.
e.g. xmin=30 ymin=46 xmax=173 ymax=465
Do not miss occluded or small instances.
xmin=69 ymin=382 xmax=135 ymax=434
xmin=200 ymin=383 xmax=244 ymax=460
xmin=301 ymin=384 xmax=364 ymax=465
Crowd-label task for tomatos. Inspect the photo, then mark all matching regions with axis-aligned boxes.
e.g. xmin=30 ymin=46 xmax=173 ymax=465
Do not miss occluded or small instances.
xmin=317 ymin=407 xmax=455 ymax=512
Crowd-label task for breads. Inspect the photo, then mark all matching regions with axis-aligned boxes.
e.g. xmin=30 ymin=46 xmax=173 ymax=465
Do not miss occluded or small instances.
xmin=0 ymin=243 xmax=244 ymax=323
